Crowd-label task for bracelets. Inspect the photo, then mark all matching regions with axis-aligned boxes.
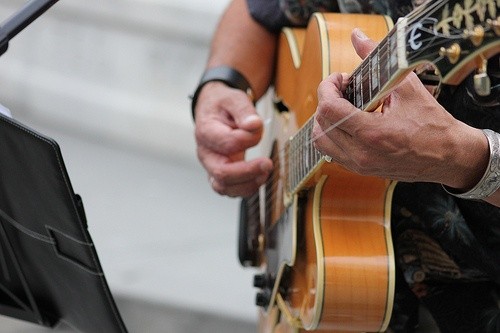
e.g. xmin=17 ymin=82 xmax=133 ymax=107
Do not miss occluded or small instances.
xmin=191 ymin=65 xmax=256 ymax=120
xmin=441 ymin=128 xmax=500 ymax=200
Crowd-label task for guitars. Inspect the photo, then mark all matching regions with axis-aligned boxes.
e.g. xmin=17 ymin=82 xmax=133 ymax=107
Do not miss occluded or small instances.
xmin=235 ymin=0 xmax=499 ymax=332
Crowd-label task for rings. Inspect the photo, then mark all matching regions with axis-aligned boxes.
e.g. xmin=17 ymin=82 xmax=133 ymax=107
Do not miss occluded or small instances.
xmin=324 ymin=154 xmax=332 ymax=163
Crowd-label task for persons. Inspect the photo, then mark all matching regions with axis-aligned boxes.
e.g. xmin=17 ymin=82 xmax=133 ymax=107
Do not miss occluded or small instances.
xmin=192 ymin=0 xmax=500 ymax=333
